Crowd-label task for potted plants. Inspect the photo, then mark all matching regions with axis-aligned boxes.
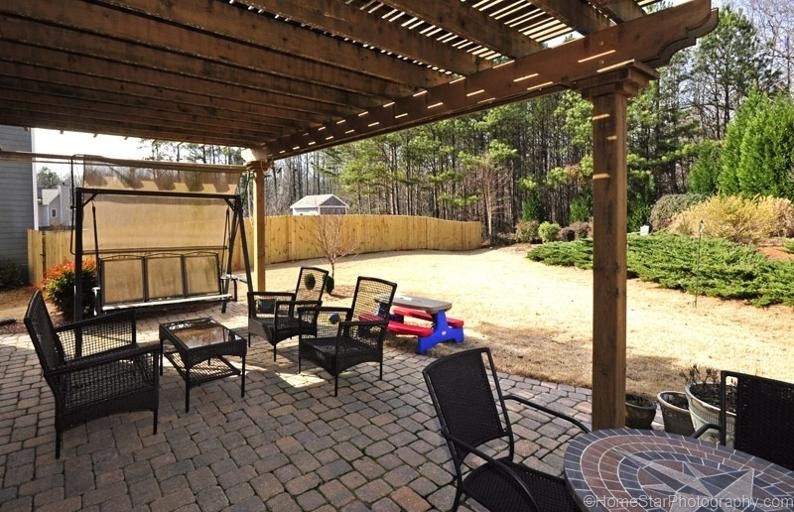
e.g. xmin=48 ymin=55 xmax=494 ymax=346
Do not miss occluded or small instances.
xmin=48 ymin=270 xmax=99 ymax=322
xmin=625 ymin=364 xmax=737 ymax=450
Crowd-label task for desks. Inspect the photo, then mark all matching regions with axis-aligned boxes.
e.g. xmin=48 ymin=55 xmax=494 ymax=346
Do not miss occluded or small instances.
xmin=374 ymin=293 xmax=465 ymax=354
xmin=561 ymin=427 xmax=794 ymax=512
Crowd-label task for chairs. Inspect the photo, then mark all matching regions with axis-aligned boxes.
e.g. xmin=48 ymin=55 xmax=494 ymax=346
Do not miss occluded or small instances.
xmin=421 ymin=346 xmax=591 ymax=512
xmin=246 ymin=266 xmax=398 ymax=398
xmin=23 ymin=288 xmax=162 ymax=460
xmin=689 ymin=370 xmax=794 ymax=471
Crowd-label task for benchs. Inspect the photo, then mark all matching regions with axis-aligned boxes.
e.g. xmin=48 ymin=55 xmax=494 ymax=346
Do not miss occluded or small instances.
xmin=360 ymin=307 xmax=465 ymax=354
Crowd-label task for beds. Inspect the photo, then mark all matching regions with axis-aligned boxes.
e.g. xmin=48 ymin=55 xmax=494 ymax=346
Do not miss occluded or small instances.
xmin=157 ymin=316 xmax=247 ymax=414
